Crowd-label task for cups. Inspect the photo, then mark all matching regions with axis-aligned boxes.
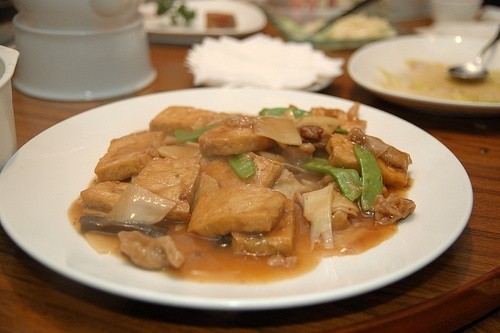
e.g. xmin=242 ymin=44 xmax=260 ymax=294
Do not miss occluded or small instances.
xmin=430 ymin=0 xmax=482 ymax=24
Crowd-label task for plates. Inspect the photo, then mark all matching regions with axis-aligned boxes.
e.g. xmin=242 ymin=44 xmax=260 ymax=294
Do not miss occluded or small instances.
xmin=138 ymin=0 xmax=268 ymax=44
xmin=347 ymin=33 xmax=500 ymax=116
xmin=267 ymin=0 xmax=397 ymax=49
xmin=0 ymin=87 xmax=474 ymax=310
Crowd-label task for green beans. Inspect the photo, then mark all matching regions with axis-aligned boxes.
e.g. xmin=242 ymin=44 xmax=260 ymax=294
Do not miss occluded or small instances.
xmin=169 ymin=107 xmax=383 ymax=215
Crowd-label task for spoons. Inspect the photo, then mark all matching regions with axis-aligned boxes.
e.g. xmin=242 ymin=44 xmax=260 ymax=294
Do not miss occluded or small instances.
xmin=449 ymin=22 xmax=500 ymax=81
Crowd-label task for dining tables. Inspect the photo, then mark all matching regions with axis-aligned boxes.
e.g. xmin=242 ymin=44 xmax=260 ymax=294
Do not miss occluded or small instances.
xmin=1 ymin=17 xmax=500 ymax=333
